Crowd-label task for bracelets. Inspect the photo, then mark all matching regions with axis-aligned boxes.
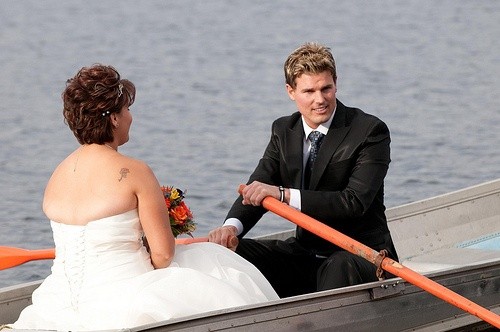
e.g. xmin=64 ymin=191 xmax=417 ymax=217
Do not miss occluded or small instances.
xmin=279 ymin=185 xmax=285 ymax=203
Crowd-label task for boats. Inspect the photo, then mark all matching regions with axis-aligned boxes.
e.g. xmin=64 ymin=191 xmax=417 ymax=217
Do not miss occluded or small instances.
xmin=0 ymin=178 xmax=500 ymax=332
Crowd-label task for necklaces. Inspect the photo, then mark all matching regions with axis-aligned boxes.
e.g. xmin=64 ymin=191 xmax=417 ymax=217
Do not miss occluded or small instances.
xmin=73 ymin=143 xmax=117 ymax=173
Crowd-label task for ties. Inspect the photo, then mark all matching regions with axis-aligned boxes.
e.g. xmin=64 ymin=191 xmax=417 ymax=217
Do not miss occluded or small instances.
xmin=304 ymin=131 xmax=324 ymax=190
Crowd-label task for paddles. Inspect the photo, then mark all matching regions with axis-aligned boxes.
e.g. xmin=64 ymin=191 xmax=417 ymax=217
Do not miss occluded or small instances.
xmin=0 ymin=235 xmax=238 ymax=271
xmin=238 ymin=184 xmax=500 ymax=328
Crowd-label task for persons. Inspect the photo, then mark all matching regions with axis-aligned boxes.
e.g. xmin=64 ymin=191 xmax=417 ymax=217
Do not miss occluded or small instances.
xmin=208 ymin=41 xmax=399 ymax=299
xmin=0 ymin=62 xmax=281 ymax=332
